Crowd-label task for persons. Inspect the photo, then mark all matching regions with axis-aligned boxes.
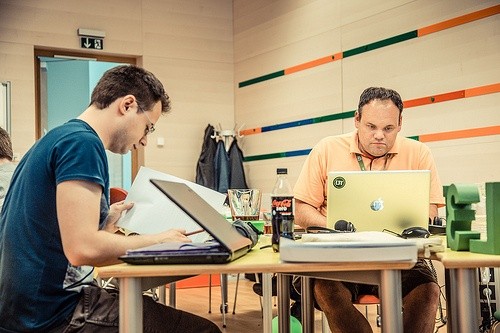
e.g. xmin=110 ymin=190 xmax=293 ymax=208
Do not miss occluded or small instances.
xmin=294 ymin=86 xmax=446 ymax=333
xmin=0 ymin=66 xmax=221 ymax=333
xmin=0 ymin=126 xmax=18 ymax=213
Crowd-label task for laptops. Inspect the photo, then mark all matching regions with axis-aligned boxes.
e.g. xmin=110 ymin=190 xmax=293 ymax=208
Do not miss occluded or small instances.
xmin=327 ymin=170 xmax=429 ymax=236
xmin=117 ymin=178 xmax=253 ymax=264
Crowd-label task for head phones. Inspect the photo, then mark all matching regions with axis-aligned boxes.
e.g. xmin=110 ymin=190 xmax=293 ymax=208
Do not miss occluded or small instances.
xmin=306 ymin=220 xmax=356 ymax=233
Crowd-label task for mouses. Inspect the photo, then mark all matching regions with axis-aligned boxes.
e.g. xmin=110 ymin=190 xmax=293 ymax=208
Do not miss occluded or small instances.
xmin=402 ymin=227 xmax=431 ymax=238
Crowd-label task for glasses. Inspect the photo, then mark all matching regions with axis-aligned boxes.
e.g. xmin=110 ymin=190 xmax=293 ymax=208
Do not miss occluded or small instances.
xmin=135 ymin=100 xmax=155 ymax=137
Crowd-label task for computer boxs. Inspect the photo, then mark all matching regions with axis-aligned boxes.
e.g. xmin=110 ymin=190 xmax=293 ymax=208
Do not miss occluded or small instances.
xmin=478 ymin=267 xmax=497 ymax=333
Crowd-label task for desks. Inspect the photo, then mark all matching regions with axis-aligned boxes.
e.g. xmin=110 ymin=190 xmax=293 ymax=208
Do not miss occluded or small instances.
xmin=93 ymin=234 xmax=500 ymax=333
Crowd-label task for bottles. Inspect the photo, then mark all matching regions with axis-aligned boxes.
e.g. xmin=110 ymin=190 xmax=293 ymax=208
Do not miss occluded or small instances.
xmin=271 ymin=167 xmax=295 ymax=253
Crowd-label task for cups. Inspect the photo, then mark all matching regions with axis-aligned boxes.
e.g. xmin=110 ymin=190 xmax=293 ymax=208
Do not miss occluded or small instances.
xmin=227 ymin=188 xmax=262 ymax=222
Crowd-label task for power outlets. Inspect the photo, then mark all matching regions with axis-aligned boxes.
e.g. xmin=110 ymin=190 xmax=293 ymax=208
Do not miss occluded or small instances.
xmin=479 ymin=284 xmax=496 ymax=300
